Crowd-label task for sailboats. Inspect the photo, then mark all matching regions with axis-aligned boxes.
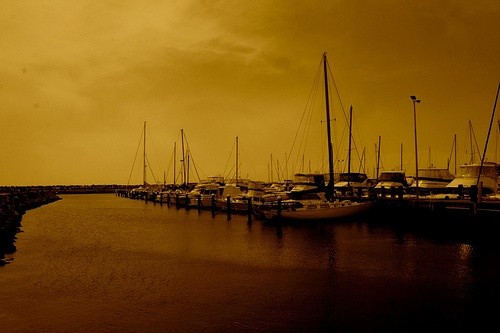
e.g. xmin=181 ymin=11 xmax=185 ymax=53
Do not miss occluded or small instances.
xmin=118 ymin=52 xmax=500 ymax=229
